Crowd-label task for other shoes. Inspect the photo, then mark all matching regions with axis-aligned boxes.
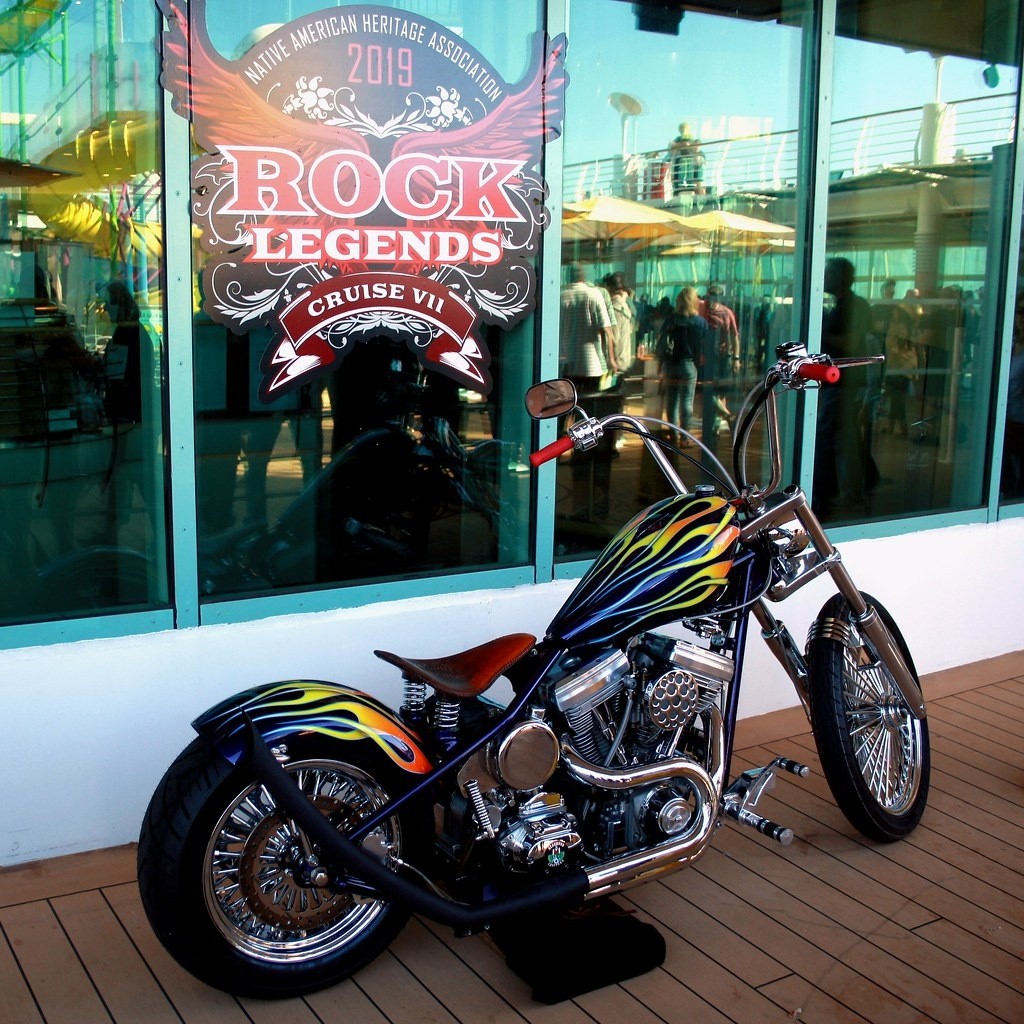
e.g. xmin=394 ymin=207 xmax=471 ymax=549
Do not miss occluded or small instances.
xmin=680 ymin=441 xmax=697 ymax=449
xmin=860 ymin=473 xmax=885 ymax=501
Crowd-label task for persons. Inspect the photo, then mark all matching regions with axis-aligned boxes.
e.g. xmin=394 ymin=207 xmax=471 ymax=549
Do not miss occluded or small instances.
xmin=89 ymin=258 xmax=982 ymax=584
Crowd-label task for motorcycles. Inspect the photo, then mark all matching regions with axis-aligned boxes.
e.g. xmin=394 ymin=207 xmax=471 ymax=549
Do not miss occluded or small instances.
xmin=24 ymin=346 xmax=581 ymax=621
xmin=133 ymin=341 xmax=933 ymax=1003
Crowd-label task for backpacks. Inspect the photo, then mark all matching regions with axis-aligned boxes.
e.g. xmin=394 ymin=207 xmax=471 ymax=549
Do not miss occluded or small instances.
xmin=662 ymin=315 xmax=698 ymax=365
